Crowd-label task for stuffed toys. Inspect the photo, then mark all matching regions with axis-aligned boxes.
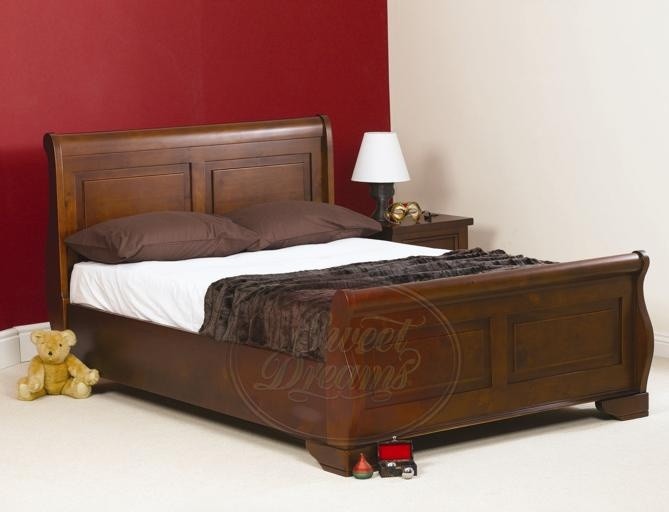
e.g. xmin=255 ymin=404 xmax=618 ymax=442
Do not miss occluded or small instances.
xmin=17 ymin=328 xmax=98 ymax=402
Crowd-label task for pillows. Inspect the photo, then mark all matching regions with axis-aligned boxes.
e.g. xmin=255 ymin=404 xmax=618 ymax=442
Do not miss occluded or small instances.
xmin=64 ymin=210 xmax=259 ymax=264
xmin=223 ymin=200 xmax=383 ymax=251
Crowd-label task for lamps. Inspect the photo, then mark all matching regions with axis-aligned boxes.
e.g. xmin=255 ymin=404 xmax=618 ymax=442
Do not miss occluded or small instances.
xmin=351 ymin=132 xmax=410 ymax=222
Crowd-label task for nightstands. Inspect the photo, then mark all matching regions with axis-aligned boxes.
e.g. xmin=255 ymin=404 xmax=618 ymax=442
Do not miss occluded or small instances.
xmin=370 ymin=213 xmax=473 ymax=250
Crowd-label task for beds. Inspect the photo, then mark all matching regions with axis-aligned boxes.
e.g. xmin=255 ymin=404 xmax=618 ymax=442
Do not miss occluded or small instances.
xmin=44 ymin=115 xmax=654 ymax=477
